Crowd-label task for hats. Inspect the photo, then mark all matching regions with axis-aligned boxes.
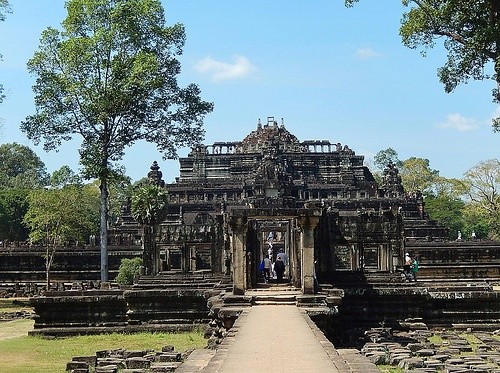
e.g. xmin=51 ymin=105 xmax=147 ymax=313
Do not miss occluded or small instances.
xmin=405 ymin=252 xmax=409 ymax=256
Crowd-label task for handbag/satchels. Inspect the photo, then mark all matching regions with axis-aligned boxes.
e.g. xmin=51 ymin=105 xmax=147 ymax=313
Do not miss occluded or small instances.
xmin=260 ymin=259 xmax=265 ymax=271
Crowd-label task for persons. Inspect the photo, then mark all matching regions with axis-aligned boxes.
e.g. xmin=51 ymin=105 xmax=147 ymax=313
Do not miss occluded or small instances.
xmin=411 ymin=258 xmax=418 ymax=282
xmin=274 ymin=257 xmax=284 ymax=282
xmin=276 ymin=249 xmax=288 ymax=272
xmin=264 ymin=255 xmax=271 ymax=283
xmin=404 ymin=253 xmax=411 ymax=272
